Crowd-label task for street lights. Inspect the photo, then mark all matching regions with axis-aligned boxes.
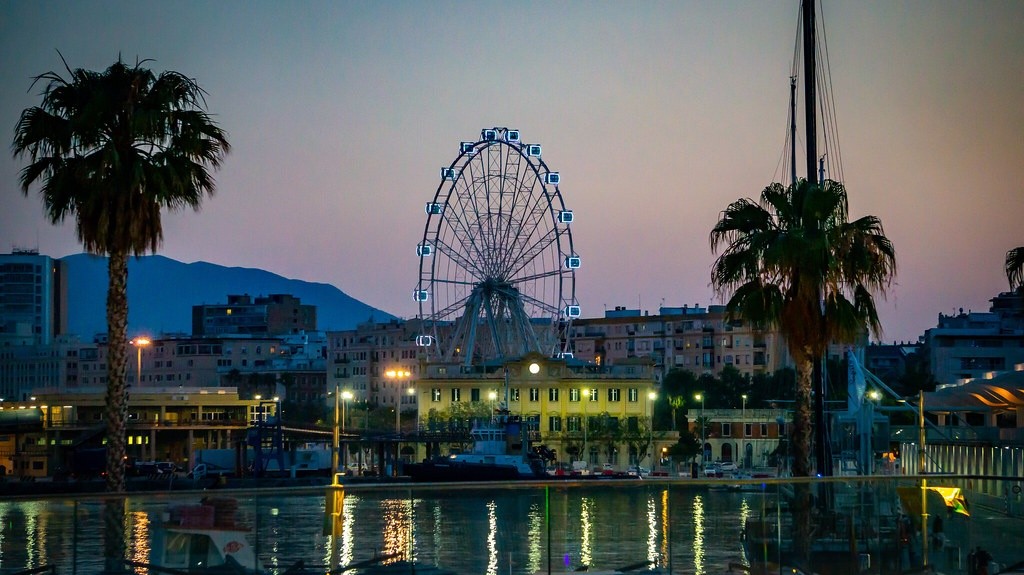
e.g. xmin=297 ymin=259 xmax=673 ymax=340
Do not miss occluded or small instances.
xmin=341 ymin=392 xmax=353 ymax=433
xmin=696 ymin=395 xmax=705 ymax=470
xmin=386 ymin=369 xmax=411 ymax=435
xmin=742 ymin=395 xmax=747 ymax=469
xmin=489 ymin=392 xmax=495 ymax=423
xmin=137 ymin=339 xmax=149 ymax=385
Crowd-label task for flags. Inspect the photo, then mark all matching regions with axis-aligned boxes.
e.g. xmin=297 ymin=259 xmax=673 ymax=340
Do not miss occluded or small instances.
xmin=937 ymin=488 xmax=971 ymax=518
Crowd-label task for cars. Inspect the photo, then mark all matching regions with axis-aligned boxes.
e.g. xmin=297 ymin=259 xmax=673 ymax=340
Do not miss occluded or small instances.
xmin=135 ymin=461 xmax=183 ymax=475
xmin=704 ymin=462 xmax=739 ymax=478
xmin=626 ymin=466 xmax=650 ymax=478
